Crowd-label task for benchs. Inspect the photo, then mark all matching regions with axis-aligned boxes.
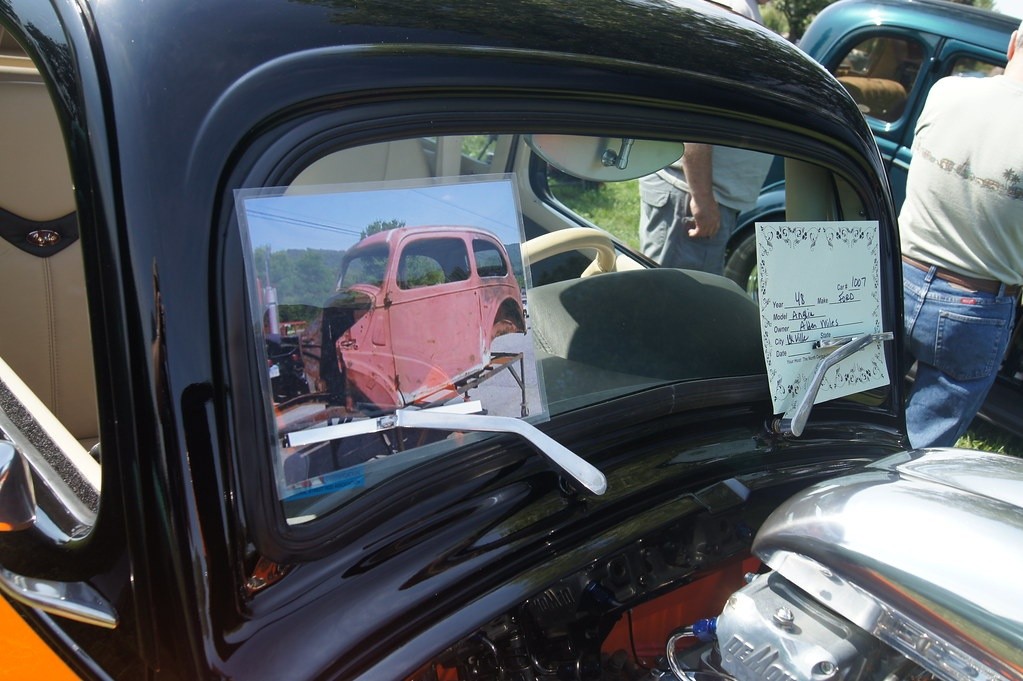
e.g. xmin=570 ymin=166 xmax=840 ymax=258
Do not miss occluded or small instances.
xmin=836 ymin=75 xmax=908 ymax=120
xmin=0 ymin=62 xmax=437 ymax=454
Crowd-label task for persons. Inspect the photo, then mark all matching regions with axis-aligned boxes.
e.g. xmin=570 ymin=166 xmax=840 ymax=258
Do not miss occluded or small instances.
xmin=897 ymin=20 xmax=1023 ymax=449
xmin=639 ymin=0 xmax=776 ymax=277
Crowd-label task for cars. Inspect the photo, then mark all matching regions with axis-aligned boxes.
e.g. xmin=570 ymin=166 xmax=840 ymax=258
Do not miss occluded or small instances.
xmin=1 ymin=1 xmax=1023 ymax=681
xmin=723 ymin=1 xmax=1022 ymax=302
xmin=298 ymin=227 xmax=526 ymax=414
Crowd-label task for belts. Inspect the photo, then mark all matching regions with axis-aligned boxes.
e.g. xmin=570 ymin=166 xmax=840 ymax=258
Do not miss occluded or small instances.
xmin=902 ymin=255 xmax=1023 ymax=297
